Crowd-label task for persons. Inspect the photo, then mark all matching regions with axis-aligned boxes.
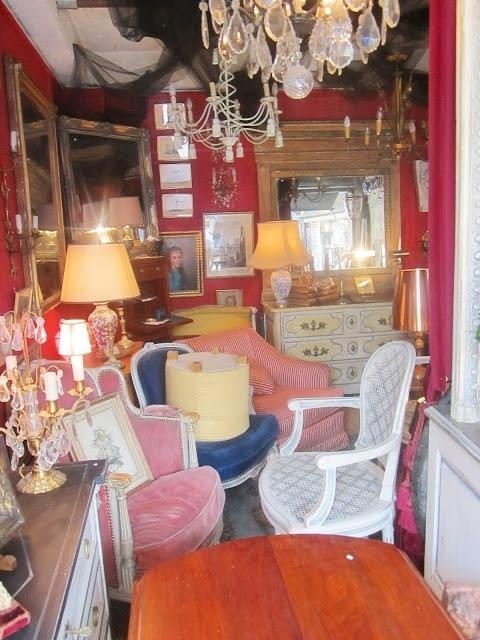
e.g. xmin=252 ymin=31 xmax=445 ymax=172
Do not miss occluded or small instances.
xmin=166 ymin=246 xmax=195 ymax=291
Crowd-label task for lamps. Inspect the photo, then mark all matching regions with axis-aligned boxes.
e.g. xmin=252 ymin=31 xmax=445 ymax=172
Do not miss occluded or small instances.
xmin=0 ymin=311 xmax=93 ymax=495
xmin=391 ymin=268 xmax=429 ymax=390
xmin=60 ymin=243 xmax=142 ymax=370
xmin=247 ymin=220 xmax=314 ymax=308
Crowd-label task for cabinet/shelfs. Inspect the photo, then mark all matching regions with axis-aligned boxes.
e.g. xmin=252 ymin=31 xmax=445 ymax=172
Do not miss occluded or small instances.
xmin=0 ymin=460 xmax=111 ymax=640
xmin=423 ymin=405 xmax=480 ymax=602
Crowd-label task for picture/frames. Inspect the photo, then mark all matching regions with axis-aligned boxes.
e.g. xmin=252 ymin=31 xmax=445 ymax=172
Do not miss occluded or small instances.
xmin=352 ymin=275 xmax=377 ymax=296
xmin=153 ymin=102 xmax=199 ymax=219
xmin=270 ymin=169 xmax=393 ymax=275
xmin=159 ymin=230 xmax=203 ymax=298
xmin=202 ymin=211 xmax=255 ymax=278
xmin=58 ymin=392 xmax=154 ymax=499
xmin=216 ymin=289 xmax=243 ymax=307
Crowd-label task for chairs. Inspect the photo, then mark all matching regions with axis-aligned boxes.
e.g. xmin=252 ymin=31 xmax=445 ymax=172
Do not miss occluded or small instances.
xmin=6 ymin=359 xmax=225 ymax=603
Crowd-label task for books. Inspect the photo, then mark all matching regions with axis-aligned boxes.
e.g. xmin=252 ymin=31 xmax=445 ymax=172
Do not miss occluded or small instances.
xmin=288 ymin=280 xmax=339 ymax=306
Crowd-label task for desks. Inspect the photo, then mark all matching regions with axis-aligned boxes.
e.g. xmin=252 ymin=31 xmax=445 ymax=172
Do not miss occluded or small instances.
xmin=128 ymin=535 xmax=469 ymax=639
